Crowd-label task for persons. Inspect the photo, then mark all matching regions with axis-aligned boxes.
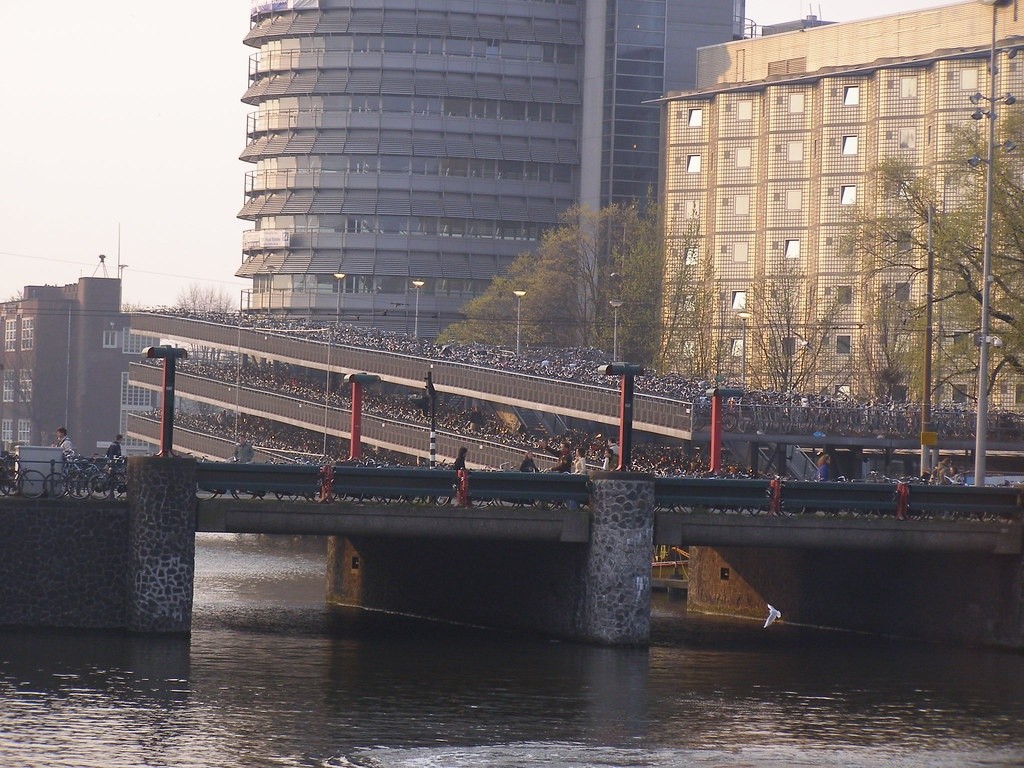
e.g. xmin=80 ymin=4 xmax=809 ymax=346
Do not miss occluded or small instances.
xmin=518 ymin=424 xmax=527 ymax=442
xmin=217 ymin=410 xmax=226 ymax=430
xmin=540 ymin=431 xmax=619 ymax=473
xmin=633 ymin=444 xmax=753 ymax=476
xmin=520 ymin=451 xmax=539 ymax=472
xmin=920 ymin=457 xmax=961 ymax=485
xmin=104 ymin=435 xmax=122 ymax=471
xmin=233 ymin=434 xmax=254 ymax=463
xmin=470 ymin=406 xmax=483 ymax=431
xmin=817 ymin=455 xmax=830 ymax=482
xmin=57 ymin=426 xmax=75 ymax=493
xmin=440 ymin=340 xmax=454 ymax=358
xmin=452 ymin=447 xmax=467 ymax=469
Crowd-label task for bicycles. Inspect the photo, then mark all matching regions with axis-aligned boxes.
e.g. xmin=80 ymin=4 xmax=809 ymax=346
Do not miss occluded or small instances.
xmin=633 ymin=371 xmax=1024 ymax=440
xmin=273 ymin=316 xmax=381 ymax=352
xmin=632 ymin=467 xmax=1023 ymax=522
xmin=143 ymin=407 xmax=227 ymax=437
xmin=132 ymin=307 xmax=273 ymax=331
xmin=542 ymin=431 xmax=773 ymax=482
xmin=283 ymin=373 xmax=411 ymax=423
xmin=228 ymin=416 xmax=328 ymax=467
xmin=141 ymin=358 xmax=283 ymax=393
xmin=384 ymin=332 xmax=620 ymax=390
xmin=328 ymin=437 xmax=428 ymax=469
xmin=412 ymin=404 xmax=542 ymax=451
xmin=0 ymin=451 xmax=586 ymax=508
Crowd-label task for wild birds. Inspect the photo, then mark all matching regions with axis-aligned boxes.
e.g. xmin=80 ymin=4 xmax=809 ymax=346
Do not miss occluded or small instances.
xmin=763 ymin=602 xmax=782 ymax=629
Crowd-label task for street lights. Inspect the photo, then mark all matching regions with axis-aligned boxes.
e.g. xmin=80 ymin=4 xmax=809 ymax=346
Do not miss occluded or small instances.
xmin=334 ymin=273 xmax=345 ymax=323
xmin=609 ymin=298 xmax=622 ymax=361
xmin=413 ymin=279 xmax=424 ymax=337
xmin=266 ymin=265 xmax=275 ymax=315
xmin=966 ymin=0 xmax=1017 ymax=483
xmin=738 ymin=310 xmax=751 ymax=386
xmin=513 ymin=289 xmax=526 ymax=354
